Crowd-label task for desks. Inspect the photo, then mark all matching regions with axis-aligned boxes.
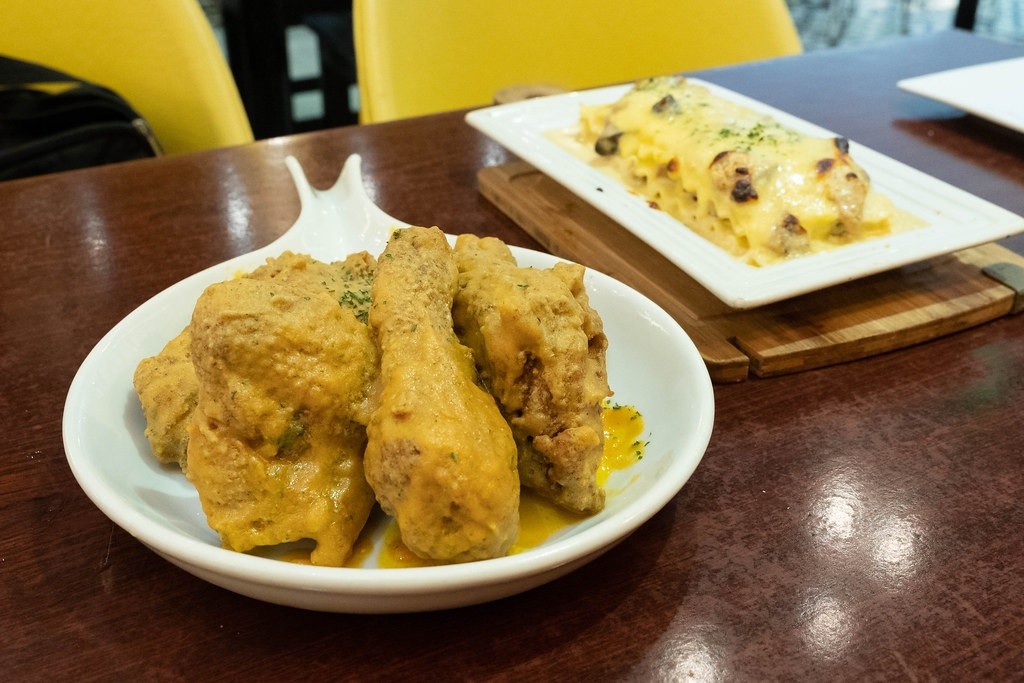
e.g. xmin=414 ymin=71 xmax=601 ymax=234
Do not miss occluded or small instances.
xmin=0 ymin=28 xmax=1024 ymax=683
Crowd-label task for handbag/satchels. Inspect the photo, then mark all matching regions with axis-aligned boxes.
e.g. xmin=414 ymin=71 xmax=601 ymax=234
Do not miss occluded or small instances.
xmin=0 ymin=55 xmax=164 ymax=182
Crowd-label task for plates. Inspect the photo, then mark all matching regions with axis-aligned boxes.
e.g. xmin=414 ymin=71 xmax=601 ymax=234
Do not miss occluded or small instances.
xmin=60 ymin=155 xmax=716 ymax=615
xmin=463 ymin=76 xmax=1024 ymax=311
xmin=897 ymin=56 xmax=1024 ymax=137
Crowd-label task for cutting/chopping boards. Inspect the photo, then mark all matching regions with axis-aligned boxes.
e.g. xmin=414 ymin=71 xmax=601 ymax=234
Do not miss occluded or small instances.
xmin=474 ymin=160 xmax=1024 ymax=383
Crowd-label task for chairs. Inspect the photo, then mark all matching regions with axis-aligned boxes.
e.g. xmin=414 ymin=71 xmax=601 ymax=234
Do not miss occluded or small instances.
xmin=352 ymin=0 xmax=802 ymax=127
xmin=0 ymin=0 xmax=257 ymax=190
